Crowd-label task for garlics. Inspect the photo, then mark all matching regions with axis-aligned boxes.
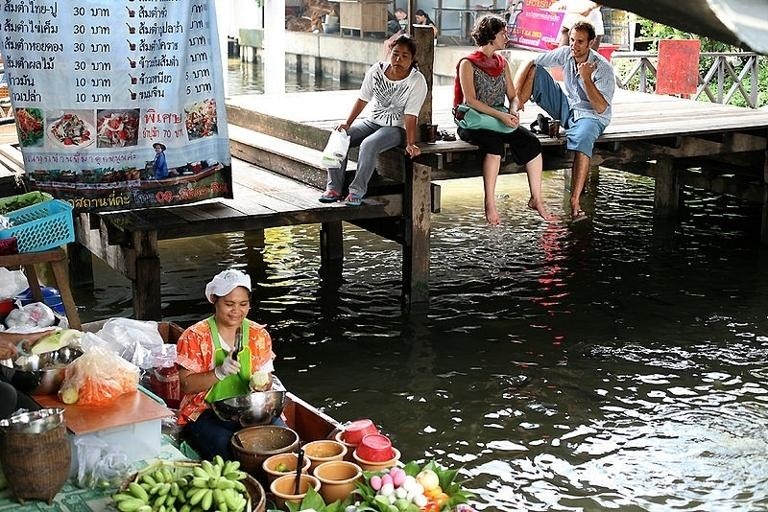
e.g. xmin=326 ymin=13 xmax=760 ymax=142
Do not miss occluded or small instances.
xmin=380 ymin=476 xmax=427 ymax=507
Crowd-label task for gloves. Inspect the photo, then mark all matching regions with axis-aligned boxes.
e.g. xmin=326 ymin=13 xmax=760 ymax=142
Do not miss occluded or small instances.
xmin=214 ymin=347 xmax=240 ymax=382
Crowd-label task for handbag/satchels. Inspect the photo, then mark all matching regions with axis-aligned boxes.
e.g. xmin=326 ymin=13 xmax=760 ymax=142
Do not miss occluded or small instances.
xmin=530 ymin=114 xmax=551 ymax=135
xmin=453 ymin=103 xmax=517 ymax=134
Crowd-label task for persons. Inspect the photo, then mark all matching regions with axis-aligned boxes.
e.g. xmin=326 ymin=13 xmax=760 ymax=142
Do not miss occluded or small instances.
xmin=175 ymin=268 xmax=277 ymax=463
xmin=633 ymin=23 xmax=658 ymax=51
xmin=556 ymin=0 xmax=605 ymax=51
xmin=512 ymin=20 xmax=615 ymax=222
xmin=318 ymin=35 xmax=429 ymax=209
xmin=415 ymin=9 xmax=438 ymax=47
xmin=452 ymin=13 xmax=554 ymax=227
xmin=143 ymin=143 xmax=169 ymax=180
xmin=394 ymin=8 xmax=408 ymax=37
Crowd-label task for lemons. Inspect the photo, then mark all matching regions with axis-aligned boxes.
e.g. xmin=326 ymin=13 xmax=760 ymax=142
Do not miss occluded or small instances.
xmin=424 ymin=487 xmax=442 ymax=498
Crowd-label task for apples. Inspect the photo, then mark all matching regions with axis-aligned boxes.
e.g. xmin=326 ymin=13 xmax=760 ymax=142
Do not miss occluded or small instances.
xmin=62 ymin=389 xmax=78 ymax=405
xmin=389 ymin=500 xmax=420 ymax=512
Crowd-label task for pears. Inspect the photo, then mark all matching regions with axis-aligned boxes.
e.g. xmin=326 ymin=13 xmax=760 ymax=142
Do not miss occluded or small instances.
xmin=416 ymin=471 xmax=439 ymax=490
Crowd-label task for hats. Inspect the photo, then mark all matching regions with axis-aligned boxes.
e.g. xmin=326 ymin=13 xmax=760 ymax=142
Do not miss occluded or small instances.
xmin=152 ymin=141 xmax=167 ymax=151
xmin=205 ymin=268 xmax=252 ymax=305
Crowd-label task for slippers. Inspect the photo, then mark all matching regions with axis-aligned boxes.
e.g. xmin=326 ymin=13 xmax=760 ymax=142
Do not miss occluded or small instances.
xmin=436 ymin=130 xmax=457 ymax=141
xmin=569 ymin=208 xmax=590 ymax=225
xmin=343 ymin=193 xmax=363 ymax=206
xmin=319 ymin=189 xmax=344 ymax=202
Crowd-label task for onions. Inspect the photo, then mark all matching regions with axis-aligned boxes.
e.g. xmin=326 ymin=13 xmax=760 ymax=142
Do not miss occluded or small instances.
xmin=370 ymin=467 xmax=406 ymax=490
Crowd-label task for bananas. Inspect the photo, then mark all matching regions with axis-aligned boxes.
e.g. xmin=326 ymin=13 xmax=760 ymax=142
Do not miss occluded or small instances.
xmin=112 ymin=455 xmax=248 ymax=512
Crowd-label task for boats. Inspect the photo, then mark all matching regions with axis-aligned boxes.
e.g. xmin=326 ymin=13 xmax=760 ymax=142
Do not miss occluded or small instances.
xmin=125 ymin=321 xmax=343 ymax=499
xmin=28 ymin=161 xmax=224 ymax=196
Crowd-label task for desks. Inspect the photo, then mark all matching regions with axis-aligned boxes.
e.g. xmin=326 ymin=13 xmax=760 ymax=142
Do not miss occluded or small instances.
xmin=0 ymin=246 xmax=84 ymax=333
xmin=15 ymin=380 xmax=176 ymax=490
xmin=338 ymin=1 xmax=392 ymax=39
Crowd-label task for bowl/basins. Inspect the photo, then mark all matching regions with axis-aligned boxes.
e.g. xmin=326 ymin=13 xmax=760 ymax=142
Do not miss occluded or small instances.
xmin=209 ymin=391 xmax=286 ymax=430
xmin=229 ymin=424 xmax=299 ymax=479
xmin=0 ymin=406 xmax=65 ymax=433
xmin=261 ymin=419 xmax=400 ymax=507
xmin=0 ymin=347 xmax=84 ymax=395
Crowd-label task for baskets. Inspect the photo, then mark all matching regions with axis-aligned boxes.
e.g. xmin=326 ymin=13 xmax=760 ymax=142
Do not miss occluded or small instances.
xmin=0 ymin=198 xmax=75 ymax=254
xmin=0 ymin=190 xmax=53 ymax=217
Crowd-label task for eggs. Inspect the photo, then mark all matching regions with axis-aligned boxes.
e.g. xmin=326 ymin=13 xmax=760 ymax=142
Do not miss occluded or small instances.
xmin=7 ymin=303 xmax=54 ymax=327
